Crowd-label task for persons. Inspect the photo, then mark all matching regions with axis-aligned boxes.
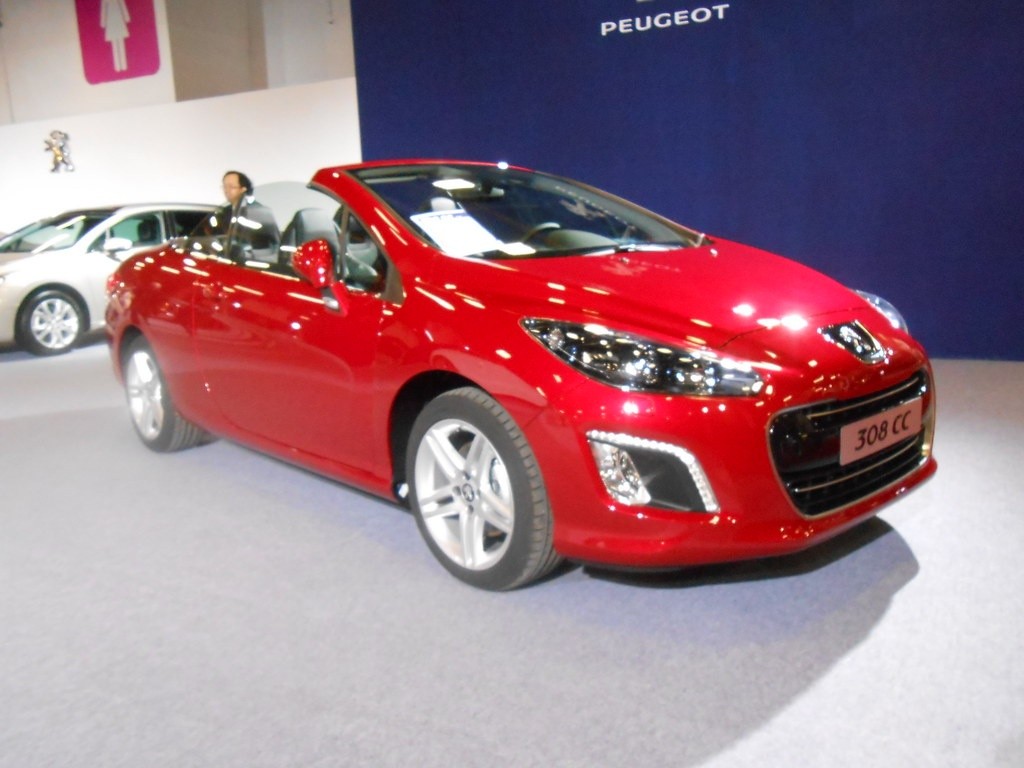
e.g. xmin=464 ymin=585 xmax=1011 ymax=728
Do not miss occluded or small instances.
xmin=214 ymin=171 xmax=279 ymax=236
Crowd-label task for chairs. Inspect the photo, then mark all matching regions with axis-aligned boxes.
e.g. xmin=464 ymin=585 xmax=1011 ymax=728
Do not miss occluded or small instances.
xmin=78 ymin=219 xmax=114 ymax=252
xmin=278 ymin=209 xmax=378 ymax=283
xmin=333 ymin=205 xmax=387 ymax=275
xmin=232 ymin=203 xmax=281 ymax=269
xmin=138 ymin=220 xmax=155 ymax=242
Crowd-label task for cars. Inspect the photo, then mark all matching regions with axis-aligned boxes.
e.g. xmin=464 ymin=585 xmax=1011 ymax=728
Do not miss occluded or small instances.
xmin=0 ymin=199 xmax=230 ymax=358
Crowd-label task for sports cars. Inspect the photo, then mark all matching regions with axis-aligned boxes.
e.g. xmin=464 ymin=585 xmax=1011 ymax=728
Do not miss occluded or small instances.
xmin=103 ymin=156 xmax=937 ymax=593
